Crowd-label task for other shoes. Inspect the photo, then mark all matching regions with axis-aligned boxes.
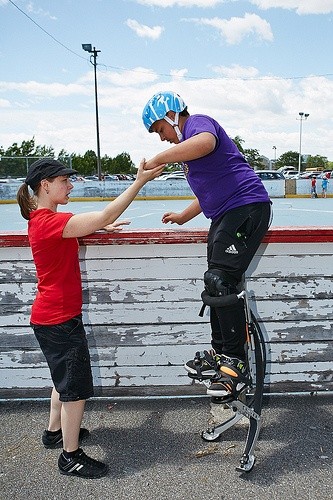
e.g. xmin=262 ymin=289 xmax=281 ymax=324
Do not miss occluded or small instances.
xmin=207 ymin=354 xmax=252 ymax=396
xmin=184 ymin=348 xmax=220 ymax=377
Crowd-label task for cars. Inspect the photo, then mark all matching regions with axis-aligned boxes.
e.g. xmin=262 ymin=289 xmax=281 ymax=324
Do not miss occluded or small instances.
xmin=1 ymin=166 xmax=333 ymax=183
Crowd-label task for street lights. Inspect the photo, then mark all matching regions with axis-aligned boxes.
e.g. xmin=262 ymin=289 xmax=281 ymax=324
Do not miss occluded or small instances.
xmin=296 ymin=112 xmax=310 ymax=173
xmin=271 ymin=146 xmax=276 ymax=160
xmin=81 ymin=43 xmax=102 ymax=180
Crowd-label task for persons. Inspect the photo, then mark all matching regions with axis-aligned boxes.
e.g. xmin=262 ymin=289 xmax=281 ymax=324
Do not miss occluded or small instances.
xmin=17 ymin=158 xmax=165 ymax=479
xmin=311 ymin=177 xmax=316 ymax=195
xmin=141 ymin=89 xmax=272 ymax=396
xmin=322 ymin=176 xmax=329 ymax=197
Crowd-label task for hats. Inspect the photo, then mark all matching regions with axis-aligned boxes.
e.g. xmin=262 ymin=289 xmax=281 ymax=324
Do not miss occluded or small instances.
xmin=28 ymin=157 xmax=77 ymax=188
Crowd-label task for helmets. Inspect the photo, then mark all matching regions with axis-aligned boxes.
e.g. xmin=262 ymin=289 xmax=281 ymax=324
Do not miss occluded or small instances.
xmin=141 ymin=90 xmax=188 ymax=133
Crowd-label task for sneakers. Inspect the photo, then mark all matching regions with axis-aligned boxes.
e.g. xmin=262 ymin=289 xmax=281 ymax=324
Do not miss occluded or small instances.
xmin=57 ymin=451 xmax=108 ymax=479
xmin=42 ymin=428 xmax=91 ymax=449
